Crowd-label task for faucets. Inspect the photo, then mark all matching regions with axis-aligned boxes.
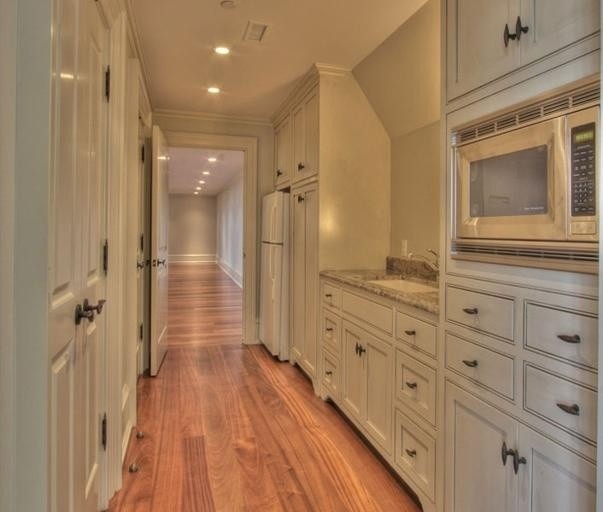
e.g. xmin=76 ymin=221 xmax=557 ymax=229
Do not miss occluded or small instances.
xmin=407 ymin=248 xmax=439 ymax=271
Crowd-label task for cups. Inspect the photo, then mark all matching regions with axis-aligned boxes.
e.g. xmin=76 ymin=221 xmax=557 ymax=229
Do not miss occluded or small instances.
xmin=401 ymin=240 xmax=408 ymax=257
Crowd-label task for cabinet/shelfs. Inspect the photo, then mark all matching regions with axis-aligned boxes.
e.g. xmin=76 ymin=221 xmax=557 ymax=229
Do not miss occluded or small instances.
xmin=273 ymin=114 xmax=290 ymax=189
xmin=0 ymin=1 xmax=110 ymax=511
xmin=289 ymin=180 xmax=318 ymax=397
xmin=341 ymin=317 xmax=391 ymax=453
xmin=440 ymin=284 xmax=597 ymax=511
xmin=446 ymin=2 xmax=602 ymax=103
xmin=291 ymin=83 xmax=317 ymax=183
xmin=391 ymin=309 xmax=437 ymax=505
xmin=319 ymin=279 xmax=340 ymax=404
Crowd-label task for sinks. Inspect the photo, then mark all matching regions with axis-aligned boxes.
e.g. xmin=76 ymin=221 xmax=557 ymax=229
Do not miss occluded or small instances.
xmin=368 ymin=279 xmax=439 ymax=293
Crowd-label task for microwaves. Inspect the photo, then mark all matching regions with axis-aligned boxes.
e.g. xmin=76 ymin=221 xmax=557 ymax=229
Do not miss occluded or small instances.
xmin=451 ymin=100 xmax=601 ymax=261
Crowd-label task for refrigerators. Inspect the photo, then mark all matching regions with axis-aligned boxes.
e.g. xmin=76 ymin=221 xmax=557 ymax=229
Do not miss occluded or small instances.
xmin=259 ymin=191 xmax=290 ymax=361
